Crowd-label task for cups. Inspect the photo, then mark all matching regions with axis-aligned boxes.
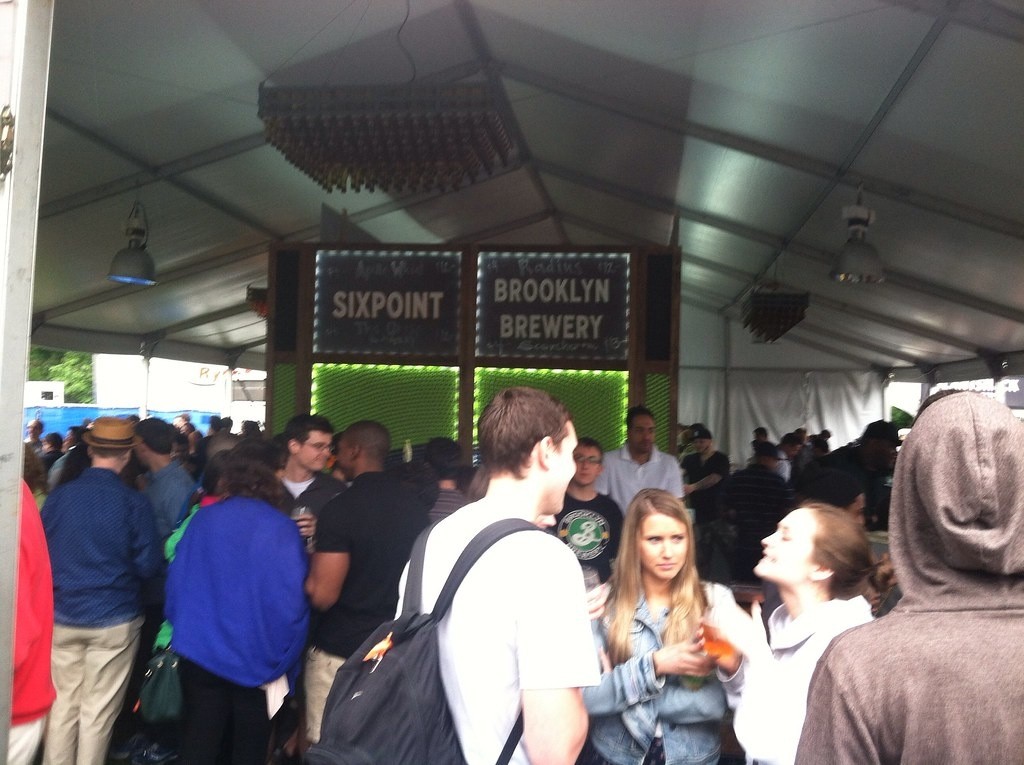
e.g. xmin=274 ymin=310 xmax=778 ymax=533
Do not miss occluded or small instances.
xmin=290 ymin=507 xmax=316 ymax=554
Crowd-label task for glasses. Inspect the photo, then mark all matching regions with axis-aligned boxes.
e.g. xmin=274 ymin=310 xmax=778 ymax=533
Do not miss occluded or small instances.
xmin=303 ymin=442 xmax=333 ymax=450
xmin=574 ymin=454 xmax=602 ymax=464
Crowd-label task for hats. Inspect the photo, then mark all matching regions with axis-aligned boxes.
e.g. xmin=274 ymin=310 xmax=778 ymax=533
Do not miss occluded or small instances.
xmin=81 ymin=416 xmax=138 ymax=449
xmin=689 ymin=429 xmax=712 ymax=441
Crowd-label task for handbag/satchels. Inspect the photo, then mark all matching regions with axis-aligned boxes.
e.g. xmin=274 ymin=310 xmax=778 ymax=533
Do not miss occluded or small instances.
xmin=133 ymin=649 xmax=185 ymax=722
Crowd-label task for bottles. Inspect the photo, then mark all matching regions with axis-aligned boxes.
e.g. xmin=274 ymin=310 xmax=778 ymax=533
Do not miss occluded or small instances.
xmin=401 ymin=440 xmax=413 ymax=463
xmin=680 ymin=605 xmax=720 ymax=691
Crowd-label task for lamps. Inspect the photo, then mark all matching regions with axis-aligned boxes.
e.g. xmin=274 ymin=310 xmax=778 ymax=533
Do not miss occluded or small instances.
xmin=246 ymin=271 xmax=269 ymax=318
xmin=830 ymin=182 xmax=886 ymax=286
xmin=257 ymin=0 xmax=523 ymax=197
xmin=108 ymin=199 xmax=158 ymax=288
xmin=739 ymin=256 xmax=810 ymax=344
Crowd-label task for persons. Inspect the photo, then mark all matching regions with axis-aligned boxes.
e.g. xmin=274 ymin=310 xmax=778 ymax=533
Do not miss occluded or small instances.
xmin=7 ymin=406 xmax=904 ymax=765
xmin=579 ymin=487 xmax=752 ymax=765
xmin=394 ymin=386 xmax=603 ymax=765
xmin=703 ymin=498 xmax=899 ymax=765
xmin=792 ymin=387 xmax=1024 ymax=765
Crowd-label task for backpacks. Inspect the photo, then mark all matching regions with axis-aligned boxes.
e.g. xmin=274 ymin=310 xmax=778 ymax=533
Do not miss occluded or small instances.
xmin=306 ymin=516 xmax=559 ymax=765
